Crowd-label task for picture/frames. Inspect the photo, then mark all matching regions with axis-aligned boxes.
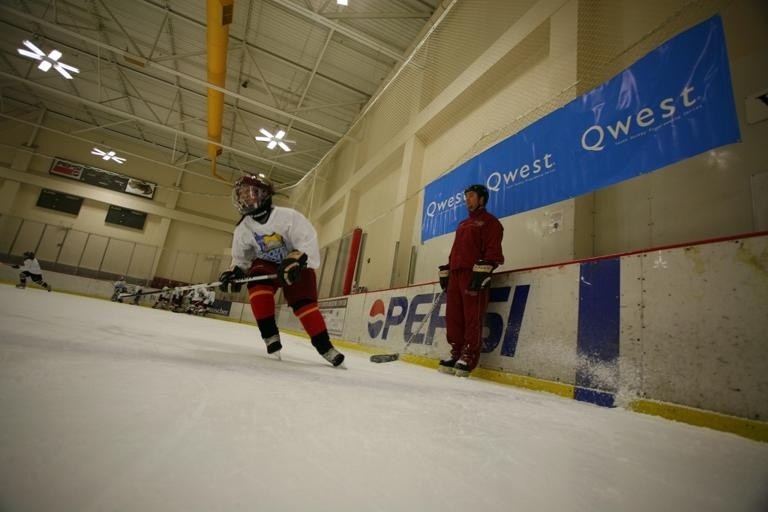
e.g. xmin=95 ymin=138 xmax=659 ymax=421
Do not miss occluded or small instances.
xmin=49 ymin=156 xmax=156 ymax=200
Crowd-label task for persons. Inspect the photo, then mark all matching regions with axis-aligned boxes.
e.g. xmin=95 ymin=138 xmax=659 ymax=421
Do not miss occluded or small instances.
xmin=12 ymin=252 xmax=52 ymax=292
xmin=438 ymin=185 xmax=505 ymax=371
xmin=219 ymin=173 xmax=345 ymax=366
xmin=111 ymin=275 xmax=216 ymax=316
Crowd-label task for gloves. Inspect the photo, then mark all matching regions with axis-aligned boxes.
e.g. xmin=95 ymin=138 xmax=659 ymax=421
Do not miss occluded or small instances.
xmin=220 ymin=265 xmax=246 ymax=293
xmin=470 ymin=262 xmax=497 ymax=290
xmin=277 ymin=250 xmax=307 ymax=282
xmin=437 ymin=264 xmax=450 ymax=288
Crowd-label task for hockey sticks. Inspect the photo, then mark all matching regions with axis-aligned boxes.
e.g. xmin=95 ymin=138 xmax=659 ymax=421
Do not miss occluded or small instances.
xmin=114 ymin=274 xmax=277 ymax=300
xmin=370 ymin=289 xmax=445 ymax=363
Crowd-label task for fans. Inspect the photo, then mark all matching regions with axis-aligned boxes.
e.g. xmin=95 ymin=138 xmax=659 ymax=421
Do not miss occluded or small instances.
xmin=90 ymin=146 xmax=127 ymax=166
xmin=17 ymin=37 xmax=81 ymax=79
xmin=254 ymin=123 xmax=297 ymax=152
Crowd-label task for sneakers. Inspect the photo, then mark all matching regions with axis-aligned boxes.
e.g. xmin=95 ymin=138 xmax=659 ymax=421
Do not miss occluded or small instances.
xmin=263 ymin=335 xmax=282 ymax=353
xmin=47 ymin=285 xmax=52 ymax=292
xmin=15 ymin=283 xmax=25 ymax=288
xmin=323 ymin=350 xmax=344 ymax=366
xmin=440 ymin=355 xmax=470 ymax=370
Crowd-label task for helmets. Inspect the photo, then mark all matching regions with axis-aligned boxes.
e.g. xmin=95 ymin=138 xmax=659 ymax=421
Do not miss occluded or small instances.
xmin=231 ymin=175 xmax=273 ymax=214
xmin=464 ymin=184 xmax=488 ymax=205
